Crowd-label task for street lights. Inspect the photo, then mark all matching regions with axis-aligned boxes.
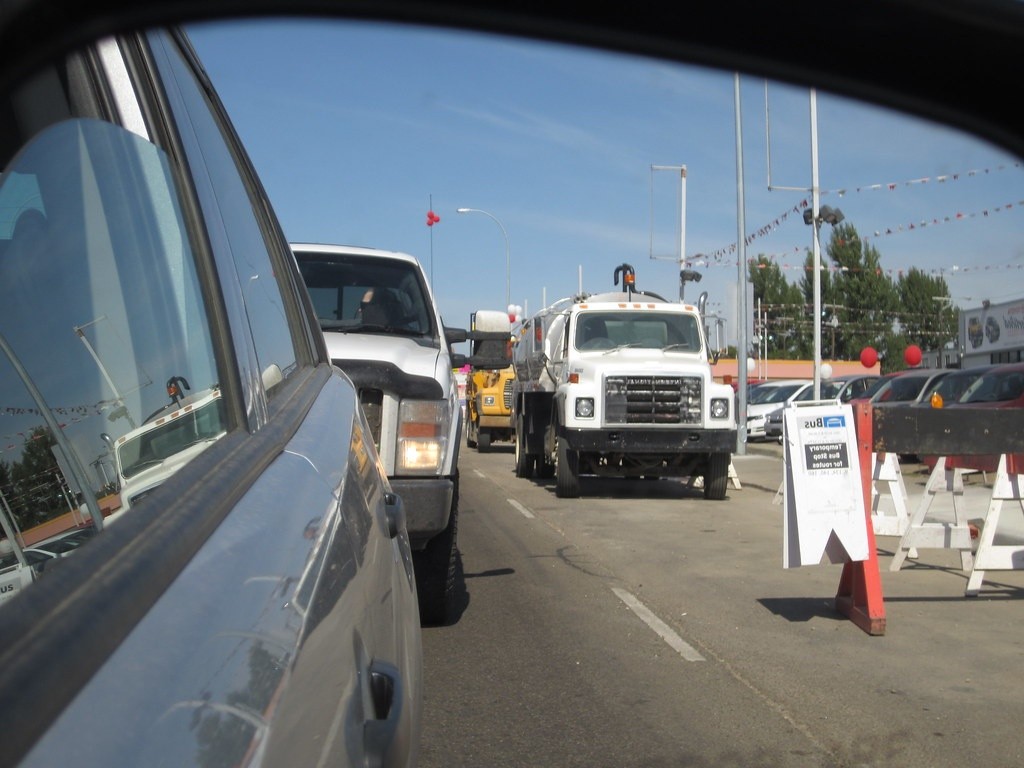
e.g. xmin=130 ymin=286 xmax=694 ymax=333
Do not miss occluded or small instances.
xmin=458 ymin=207 xmax=511 ymax=314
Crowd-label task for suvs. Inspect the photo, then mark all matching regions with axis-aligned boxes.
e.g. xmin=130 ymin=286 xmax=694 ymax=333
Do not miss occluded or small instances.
xmin=283 ymin=236 xmax=472 ymax=608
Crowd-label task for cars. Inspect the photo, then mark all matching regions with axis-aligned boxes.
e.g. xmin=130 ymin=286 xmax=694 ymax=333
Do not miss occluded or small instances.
xmin=732 ymin=367 xmax=1023 ymax=474
xmin=1 ymin=0 xmax=421 ymax=767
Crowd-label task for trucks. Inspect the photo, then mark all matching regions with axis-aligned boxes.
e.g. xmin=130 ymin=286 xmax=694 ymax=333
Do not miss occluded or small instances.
xmin=510 ymin=284 xmax=739 ymax=500
xmin=466 ymin=352 xmax=517 ymax=453
